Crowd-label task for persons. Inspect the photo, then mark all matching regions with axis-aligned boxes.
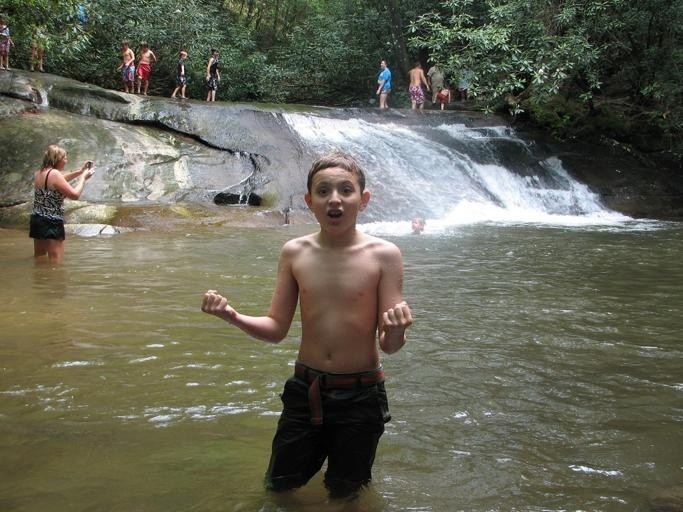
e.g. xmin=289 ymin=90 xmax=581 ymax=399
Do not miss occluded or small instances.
xmin=29 ymin=145 xmax=97 ymax=259
xmin=433 ymin=84 xmax=450 ymax=110
xmin=206 ymin=48 xmax=220 ymax=101
xmin=30 ymin=43 xmax=45 ymax=72
xmin=411 ymin=217 xmax=424 ymax=234
xmin=171 ymin=51 xmax=189 ymax=99
xmin=376 ymin=60 xmax=392 ymax=108
xmin=0 ymin=20 xmax=13 ymax=71
xmin=408 ymin=63 xmax=430 ymax=110
xmin=201 ymin=152 xmax=414 ymax=498
xmin=117 ymin=41 xmax=135 ymax=94
xmin=136 ymin=42 xmax=156 ymax=95
xmin=427 ymin=66 xmax=444 ymax=104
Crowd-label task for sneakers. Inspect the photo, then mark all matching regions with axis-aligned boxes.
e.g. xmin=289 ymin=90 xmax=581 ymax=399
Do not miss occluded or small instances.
xmin=38 ymin=67 xmax=46 ymax=72
xmin=30 ymin=65 xmax=35 ymax=72
xmin=0 ymin=65 xmax=11 ymax=71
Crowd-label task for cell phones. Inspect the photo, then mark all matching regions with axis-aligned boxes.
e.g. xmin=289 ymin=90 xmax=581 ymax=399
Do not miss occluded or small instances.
xmin=88 ymin=162 xmax=93 ymax=169
